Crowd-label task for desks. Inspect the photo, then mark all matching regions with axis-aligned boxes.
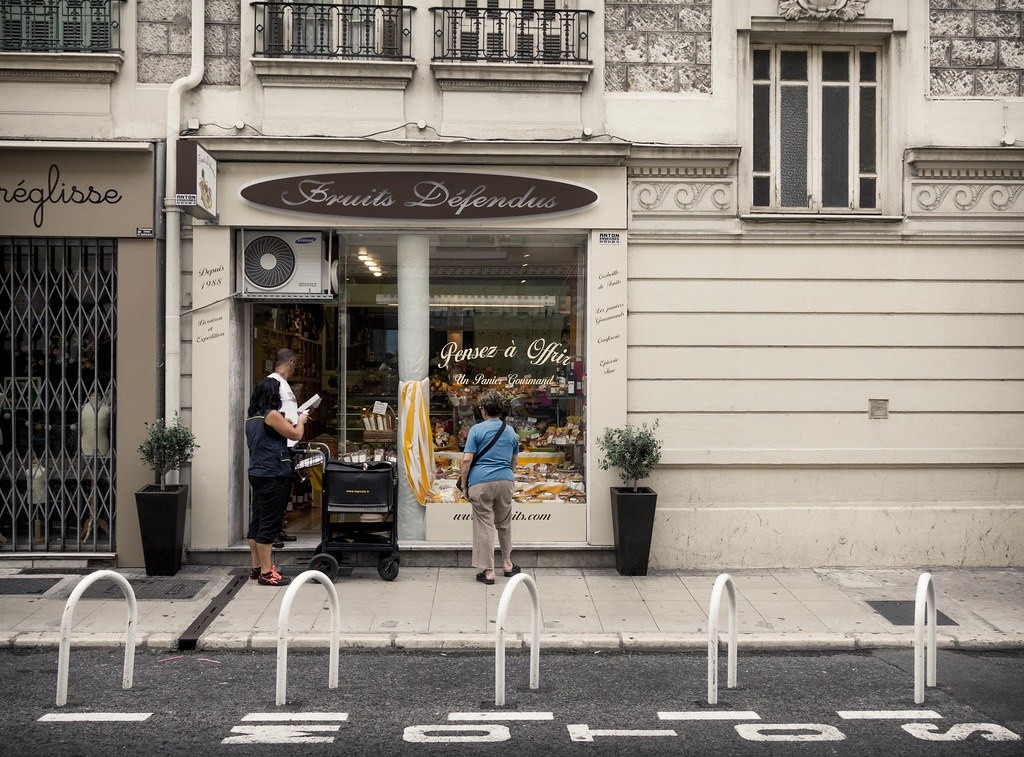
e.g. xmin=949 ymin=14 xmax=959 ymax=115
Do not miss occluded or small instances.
xmin=0 ymin=457 xmax=115 ymax=544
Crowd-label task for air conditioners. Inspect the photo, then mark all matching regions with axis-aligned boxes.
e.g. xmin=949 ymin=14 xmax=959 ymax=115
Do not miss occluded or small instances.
xmin=236 ymin=230 xmax=331 ymax=293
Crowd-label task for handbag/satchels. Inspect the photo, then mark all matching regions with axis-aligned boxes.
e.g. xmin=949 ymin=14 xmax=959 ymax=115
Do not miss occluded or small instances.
xmin=456 ymin=475 xmax=463 ymax=491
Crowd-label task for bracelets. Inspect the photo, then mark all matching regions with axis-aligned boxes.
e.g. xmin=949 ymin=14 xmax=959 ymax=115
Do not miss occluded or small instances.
xmin=462 ymin=486 xmax=467 ymax=491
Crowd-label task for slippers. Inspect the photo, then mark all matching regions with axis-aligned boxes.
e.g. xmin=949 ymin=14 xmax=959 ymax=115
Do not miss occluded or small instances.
xmin=504 ymin=562 xmax=521 ymax=576
xmin=476 ymin=569 xmax=495 ymax=584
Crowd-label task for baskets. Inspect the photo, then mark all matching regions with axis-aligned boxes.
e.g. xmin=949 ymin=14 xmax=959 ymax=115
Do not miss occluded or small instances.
xmin=364 ymin=403 xmax=397 ymax=441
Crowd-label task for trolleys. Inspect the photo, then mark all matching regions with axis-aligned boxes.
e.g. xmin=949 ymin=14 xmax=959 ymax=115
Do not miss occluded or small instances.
xmin=291 ymin=441 xmax=402 ymax=584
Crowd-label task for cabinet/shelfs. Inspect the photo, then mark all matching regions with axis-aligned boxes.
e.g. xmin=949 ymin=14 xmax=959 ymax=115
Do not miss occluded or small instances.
xmin=254 ymin=303 xmax=586 ymax=512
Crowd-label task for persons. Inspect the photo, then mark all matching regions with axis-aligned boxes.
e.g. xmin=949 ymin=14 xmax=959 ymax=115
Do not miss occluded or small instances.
xmin=263 ymin=347 xmax=310 ymax=548
xmin=26 ymin=462 xmax=49 ymax=504
xmin=80 ymin=393 xmax=111 ymax=456
xmin=379 ymin=352 xmax=395 ymax=372
xmin=244 ymin=378 xmax=308 ymax=586
xmin=460 ymin=388 xmax=520 ymax=583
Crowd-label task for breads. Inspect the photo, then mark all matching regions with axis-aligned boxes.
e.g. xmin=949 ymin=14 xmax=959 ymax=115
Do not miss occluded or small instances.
xmin=342 ymin=412 xmax=398 ymax=479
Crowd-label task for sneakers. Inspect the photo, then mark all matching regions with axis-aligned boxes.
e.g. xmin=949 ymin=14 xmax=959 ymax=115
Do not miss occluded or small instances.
xmin=251 ymin=567 xmax=282 ymax=579
xmin=256 ymin=565 xmax=291 ymax=585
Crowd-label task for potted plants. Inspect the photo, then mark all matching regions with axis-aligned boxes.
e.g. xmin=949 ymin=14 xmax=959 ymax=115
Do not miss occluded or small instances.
xmin=134 ymin=410 xmax=201 ymax=578
xmin=595 ymin=416 xmax=663 ymax=576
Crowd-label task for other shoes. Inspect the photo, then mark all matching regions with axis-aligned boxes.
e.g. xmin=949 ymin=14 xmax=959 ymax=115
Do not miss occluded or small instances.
xmin=272 ymin=538 xmax=284 ymax=547
xmin=279 ymin=530 xmax=297 ymax=541
